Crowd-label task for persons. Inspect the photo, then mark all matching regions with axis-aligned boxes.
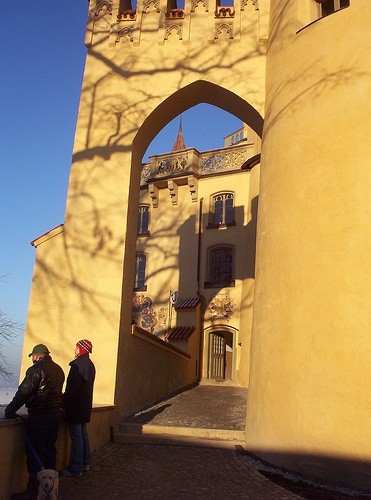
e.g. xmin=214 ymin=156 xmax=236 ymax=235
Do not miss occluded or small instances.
xmin=59 ymin=339 xmax=96 ymax=476
xmin=5 ymin=344 xmax=65 ymax=500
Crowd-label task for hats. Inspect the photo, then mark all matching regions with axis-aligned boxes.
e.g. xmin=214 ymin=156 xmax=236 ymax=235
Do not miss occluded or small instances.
xmin=29 ymin=344 xmax=50 ymax=357
xmin=76 ymin=339 xmax=92 ymax=357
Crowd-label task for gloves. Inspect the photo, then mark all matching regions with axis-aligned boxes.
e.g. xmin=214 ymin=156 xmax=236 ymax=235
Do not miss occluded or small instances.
xmin=5 ymin=413 xmax=19 ymax=418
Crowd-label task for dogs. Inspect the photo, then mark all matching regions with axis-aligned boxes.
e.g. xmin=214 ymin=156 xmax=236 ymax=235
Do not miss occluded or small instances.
xmin=37 ymin=469 xmax=58 ymax=500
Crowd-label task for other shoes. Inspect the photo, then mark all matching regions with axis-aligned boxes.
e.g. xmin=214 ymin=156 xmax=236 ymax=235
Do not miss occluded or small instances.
xmin=83 ymin=467 xmax=90 ymax=471
xmin=62 ymin=468 xmax=82 ymax=476
xmin=10 ymin=492 xmax=37 ymax=500
